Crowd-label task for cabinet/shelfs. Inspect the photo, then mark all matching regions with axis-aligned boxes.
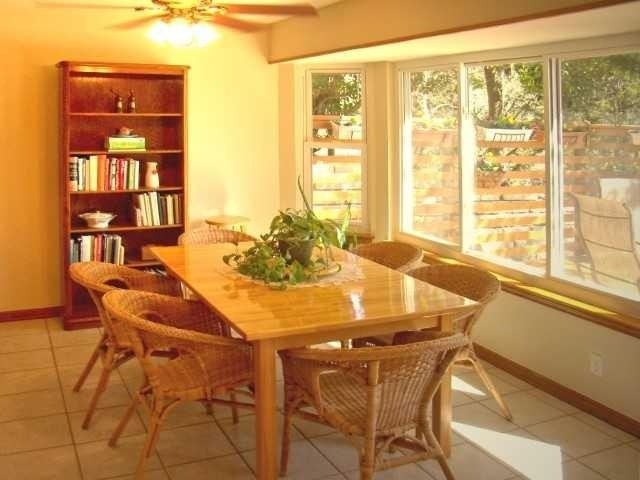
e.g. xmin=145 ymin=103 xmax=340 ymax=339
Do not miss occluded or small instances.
xmin=313 ymin=115 xmax=639 ymax=265
xmin=57 ymin=60 xmax=191 ymax=331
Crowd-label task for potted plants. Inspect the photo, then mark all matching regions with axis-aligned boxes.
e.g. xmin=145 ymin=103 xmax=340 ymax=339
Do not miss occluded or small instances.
xmin=331 ymin=115 xmax=591 ymax=147
xmin=222 ymin=174 xmax=357 ymax=290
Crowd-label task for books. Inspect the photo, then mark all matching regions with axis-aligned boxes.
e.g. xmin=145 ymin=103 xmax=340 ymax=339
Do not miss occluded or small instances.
xmin=68 ymin=136 xmax=181 ymax=275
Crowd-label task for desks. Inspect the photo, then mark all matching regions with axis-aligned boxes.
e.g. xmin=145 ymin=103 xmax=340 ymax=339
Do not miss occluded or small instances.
xmin=148 ymin=239 xmax=482 ymax=479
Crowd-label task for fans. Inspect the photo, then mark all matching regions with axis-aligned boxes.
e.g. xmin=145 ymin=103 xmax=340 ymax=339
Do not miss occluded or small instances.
xmin=108 ymin=0 xmax=317 ymax=50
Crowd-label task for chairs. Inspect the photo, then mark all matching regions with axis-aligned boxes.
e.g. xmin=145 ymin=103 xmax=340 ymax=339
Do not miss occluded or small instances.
xmin=352 ymin=265 xmax=512 ymax=421
xmin=351 ymin=240 xmax=424 ymax=273
xmin=278 ymin=329 xmax=468 ymax=480
xmin=102 ymin=289 xmax=254 ymax=480
xmin=177 ymin=230 xmax=257 ymax=245
xmin=564 ymin=178 xmax=640 ymax=295
xmin=68 ymin=261 xmax=184 ymax=431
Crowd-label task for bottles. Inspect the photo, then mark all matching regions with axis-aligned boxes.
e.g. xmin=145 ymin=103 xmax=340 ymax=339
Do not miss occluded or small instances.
xmin=144 ymin=161 xmax=160 ymax=188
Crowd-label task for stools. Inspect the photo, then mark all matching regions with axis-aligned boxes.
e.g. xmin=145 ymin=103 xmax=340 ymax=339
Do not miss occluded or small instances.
xmin=205 ymin=215 xmax=251 ymax=232
